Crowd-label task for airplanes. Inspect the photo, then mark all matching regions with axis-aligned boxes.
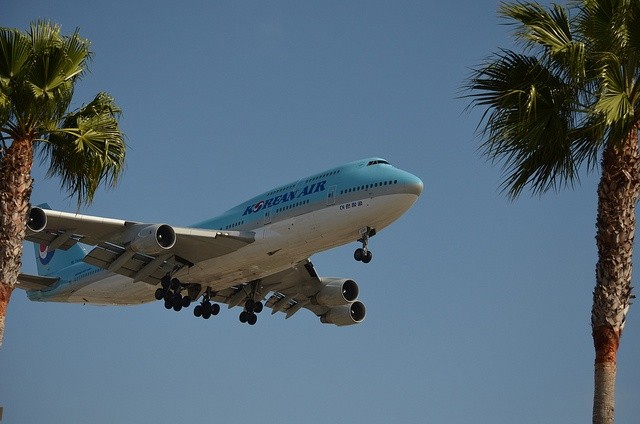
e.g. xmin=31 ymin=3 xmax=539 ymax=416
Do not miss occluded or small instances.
xmin=15 ymin=157 xmax=424 ymax=326
